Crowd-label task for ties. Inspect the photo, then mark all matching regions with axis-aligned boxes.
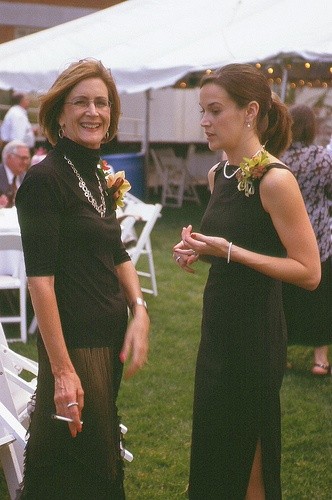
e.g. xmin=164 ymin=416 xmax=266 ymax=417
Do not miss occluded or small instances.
xmin=8 ymin=175 xmax=18 ymax=202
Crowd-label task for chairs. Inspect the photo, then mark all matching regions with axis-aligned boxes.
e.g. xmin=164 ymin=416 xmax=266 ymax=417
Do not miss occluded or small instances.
xmin=0 ymin=201 xmax=163 ymax=500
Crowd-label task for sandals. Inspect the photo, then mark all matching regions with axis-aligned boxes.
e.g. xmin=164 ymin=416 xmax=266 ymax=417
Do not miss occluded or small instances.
xmin=312 ymin=361 xmax=330 ymax=376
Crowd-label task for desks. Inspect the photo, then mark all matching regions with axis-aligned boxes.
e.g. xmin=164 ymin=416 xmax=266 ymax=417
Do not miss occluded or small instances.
xmin=0 ymin=206 xmax=46 ymax=283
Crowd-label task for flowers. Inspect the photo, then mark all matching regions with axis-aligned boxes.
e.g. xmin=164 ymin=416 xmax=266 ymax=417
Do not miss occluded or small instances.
xmin=98 ymin=162 xmax=134 ymax=212
xmin=235 ymin=153 xmax=272 ymax=196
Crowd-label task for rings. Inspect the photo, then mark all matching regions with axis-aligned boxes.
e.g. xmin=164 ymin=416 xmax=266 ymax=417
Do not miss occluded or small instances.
xmin=175 ymin=256 xmax=181 ymax=263
xmin=66 ymin=402 xmax=80 ymax=409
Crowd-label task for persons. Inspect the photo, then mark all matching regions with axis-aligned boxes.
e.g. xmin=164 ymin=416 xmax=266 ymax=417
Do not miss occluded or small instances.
xmin=0 ymin=140 xmax=30 ymax=210
xmin=171 ymin=62 xmax=322 ymax=500
xmin=0 ymin=90 xmax=35 ymax=161
xmin=13 ymin=57 xmax=151 ymax=500
xmin=277 ymin=104 xmax=332 ymax=375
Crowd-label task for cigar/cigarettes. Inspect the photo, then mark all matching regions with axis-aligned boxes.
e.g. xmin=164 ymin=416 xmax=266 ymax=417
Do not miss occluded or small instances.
xmin=52 ymin=414 xmax=84 ymax=425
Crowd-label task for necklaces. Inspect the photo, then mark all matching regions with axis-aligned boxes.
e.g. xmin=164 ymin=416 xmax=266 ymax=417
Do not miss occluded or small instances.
xmin=223 ymin=143 xmax=265 ymax=180
xmin=63 ymin=155 xmax=107 ymax=219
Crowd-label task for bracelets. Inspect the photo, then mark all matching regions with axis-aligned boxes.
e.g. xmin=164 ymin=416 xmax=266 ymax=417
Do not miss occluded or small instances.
xmin=227 ymin=243 xmax=232 ymax=265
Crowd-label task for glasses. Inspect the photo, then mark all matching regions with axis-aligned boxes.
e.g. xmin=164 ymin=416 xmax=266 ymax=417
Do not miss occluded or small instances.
xmin=12 ymin=153 xmax=30 ymax=162
xmin=62 ymin=97 xmax=113 ymax=112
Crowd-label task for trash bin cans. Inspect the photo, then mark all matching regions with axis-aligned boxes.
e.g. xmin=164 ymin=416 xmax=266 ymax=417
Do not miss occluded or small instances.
xmin=100 ymin=153 xmax=146 ymax=204
